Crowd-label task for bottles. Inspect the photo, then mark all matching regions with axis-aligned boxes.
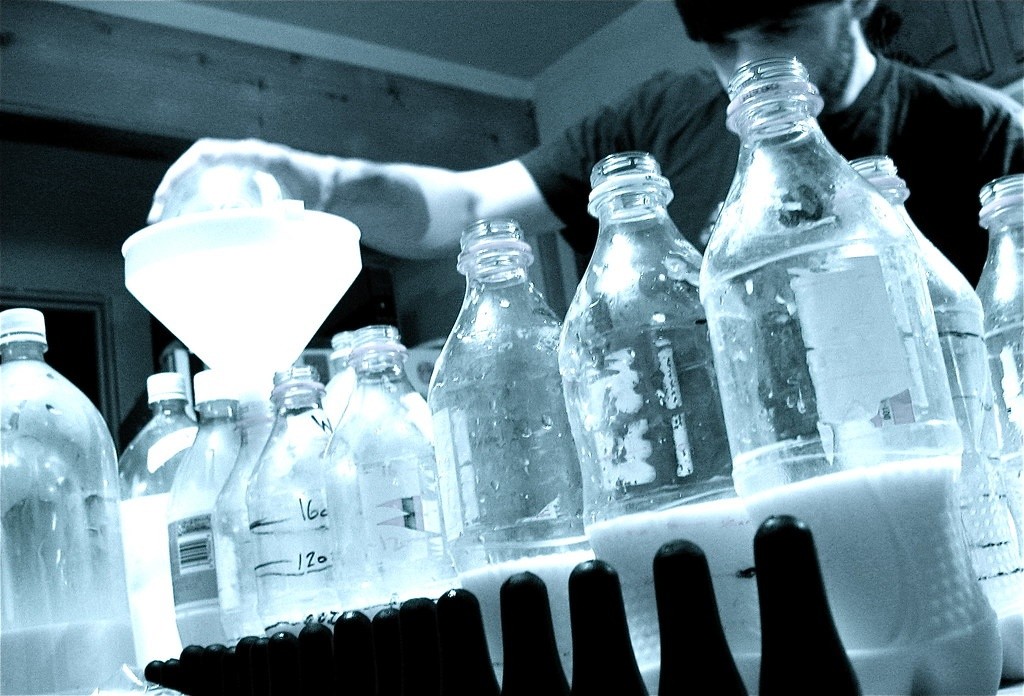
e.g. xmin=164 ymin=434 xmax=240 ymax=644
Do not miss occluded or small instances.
xmin=556 ymin=151 xmax=778 ymax=696
xmin=831 ymin=152 xmax=1024 ymax=680
xmin=169 ymin=321 xmax=447 ymax=653
xmin=425 ymin=217 xmax=597 ymax=689
xmin=113 ymin=371 xmax=207 ymax=673
xmin=974 ymin=168 xmax=1024 ymax=534
xmin=0 ymin=308 xmax=141 ymax=696
xmin=698 ymin=54 xmax=1005 ymax=693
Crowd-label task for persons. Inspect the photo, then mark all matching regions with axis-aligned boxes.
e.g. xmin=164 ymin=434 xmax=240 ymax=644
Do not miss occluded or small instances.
xmin=147 ymin=0 xmax=1023 ymax=289
